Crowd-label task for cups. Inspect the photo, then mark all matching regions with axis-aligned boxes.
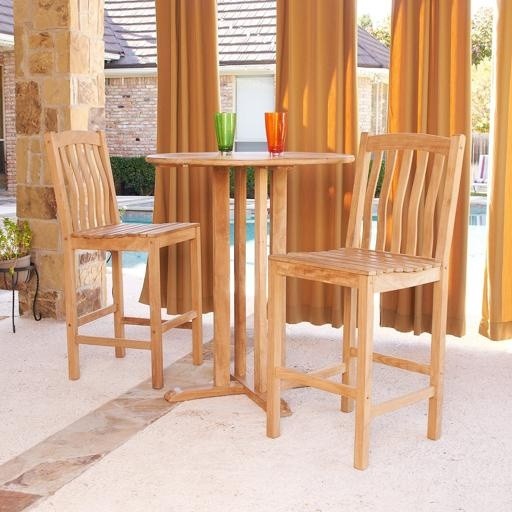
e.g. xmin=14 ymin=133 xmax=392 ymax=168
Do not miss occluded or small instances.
xmin=215 ymin=113 xmax=237 ymax=154
xmin=265 ymin=112 xmax=288 ymax=155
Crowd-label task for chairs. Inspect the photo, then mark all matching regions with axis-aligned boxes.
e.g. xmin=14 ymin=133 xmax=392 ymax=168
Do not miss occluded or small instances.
xmin=42 ymin=128 xmax=204 ymax=390
xmin=263 ymin=132 xmax=467 ymax=470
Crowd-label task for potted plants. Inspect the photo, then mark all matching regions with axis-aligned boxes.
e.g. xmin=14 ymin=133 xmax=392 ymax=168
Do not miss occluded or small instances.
xmin=0 ymin=218 xmax=31 ymax=287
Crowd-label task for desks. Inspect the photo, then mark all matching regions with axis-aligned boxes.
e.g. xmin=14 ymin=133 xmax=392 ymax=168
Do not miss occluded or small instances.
xmin=146 ymin=151 xmax=358 ymax=419
